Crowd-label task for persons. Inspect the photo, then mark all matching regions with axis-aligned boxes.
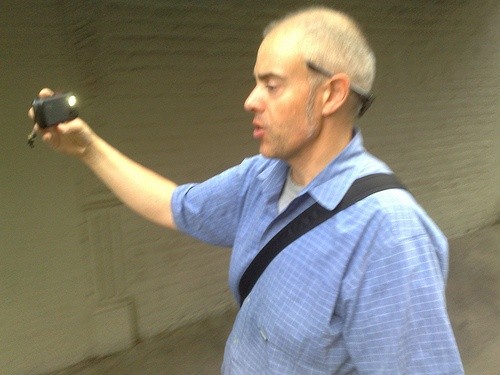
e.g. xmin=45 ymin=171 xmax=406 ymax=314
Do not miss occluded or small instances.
xmin=29 ymin=7 xmax=464 ymax=375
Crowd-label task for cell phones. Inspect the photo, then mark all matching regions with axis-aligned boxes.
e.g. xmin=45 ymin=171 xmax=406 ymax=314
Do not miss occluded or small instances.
xmin=33 ymin=92 xmax=79 ymax=128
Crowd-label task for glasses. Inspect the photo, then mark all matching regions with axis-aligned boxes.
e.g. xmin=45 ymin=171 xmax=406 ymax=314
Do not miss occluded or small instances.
xmin=308 ymin=62 xmax=375 ymax=118
